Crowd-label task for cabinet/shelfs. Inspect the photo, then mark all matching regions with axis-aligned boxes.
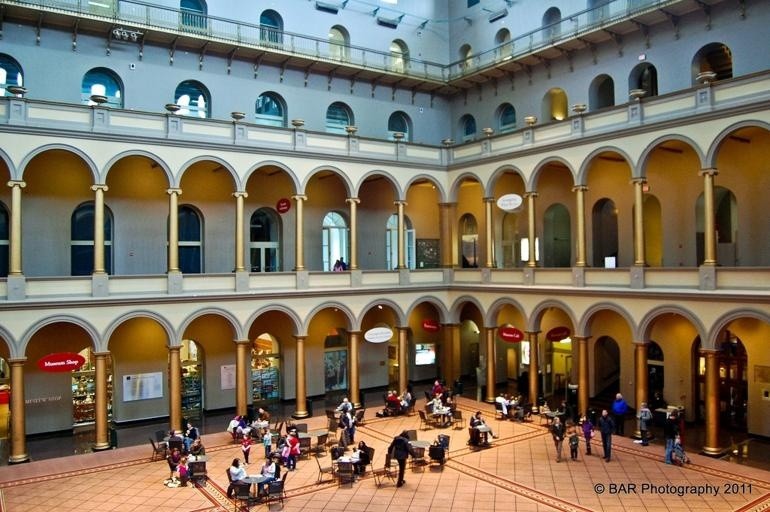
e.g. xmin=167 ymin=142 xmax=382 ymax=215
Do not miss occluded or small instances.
xmin=71 ymin=370 xmax=113 ymax=423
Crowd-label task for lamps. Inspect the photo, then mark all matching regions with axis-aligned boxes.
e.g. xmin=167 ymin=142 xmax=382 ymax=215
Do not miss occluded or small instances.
xmin=498 ymin=193 xmax=526 ymax=216
xmin=364 ymin=304 xmax=393 ymax=344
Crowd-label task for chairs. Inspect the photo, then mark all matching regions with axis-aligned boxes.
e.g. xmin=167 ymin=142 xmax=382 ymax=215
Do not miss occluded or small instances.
xmin=492 ymin=398 xmax=567 ymax=429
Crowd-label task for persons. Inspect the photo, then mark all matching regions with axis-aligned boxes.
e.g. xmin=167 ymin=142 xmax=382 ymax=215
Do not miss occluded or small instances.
xmin=339 ymin=258 xmax=346 ymax=270
xmin=330 ymin=397 xmax=369 ymax=474
xmin=163 ymin=424 xmax=205 ymax=486
xmin=495 ymin=391 xmax=529 ymax=421
xmin=661 ymin=413 xmax=678 ymax=466
xmin=386 ymin=379 xmax=456 ymax=426
xmin=540 ymin=393 xmax=628 ymax=462
xmin=334 ymin=260 xmax=343 ymax=272
xmin=653 ymin=391 xmax=663 ymax=408
xmin=388 ymin=430 xmax=416 ymax=487
xmin=226 ymin=408 xmax=300 ymax=497
xmin=639 ymin=402 xmax=652 ymax=447
xmin=470 ymin=411 xmax=498 ymax=446
